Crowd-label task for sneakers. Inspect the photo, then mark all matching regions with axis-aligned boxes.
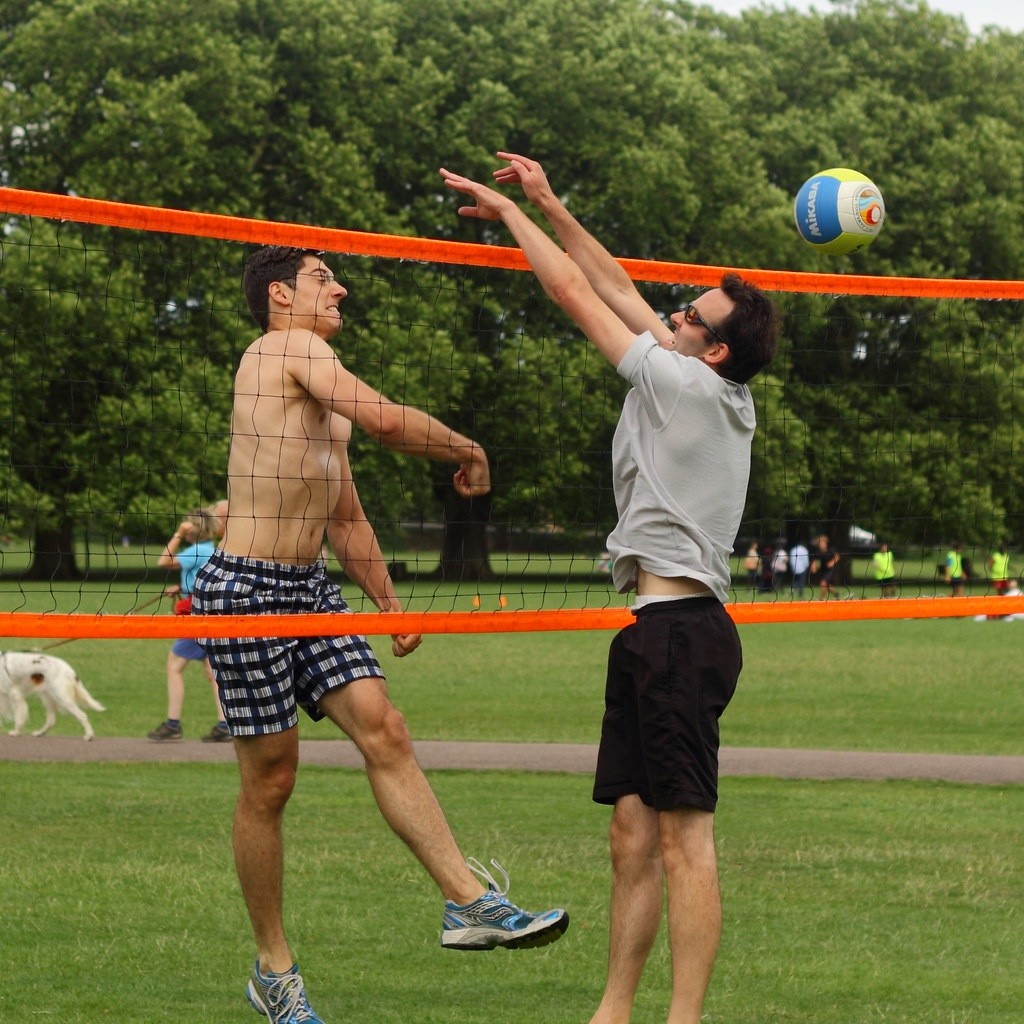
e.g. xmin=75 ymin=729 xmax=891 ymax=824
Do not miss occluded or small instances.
xmin=443 ymin=857 xmax=569 ymax=951
xmin=199 ymin=726 xmax=232 ymax=741
xmin=242 ymin=960 xmax=324 ymax=1024
xmin=148 ymin=723 xmax=182 ymax=743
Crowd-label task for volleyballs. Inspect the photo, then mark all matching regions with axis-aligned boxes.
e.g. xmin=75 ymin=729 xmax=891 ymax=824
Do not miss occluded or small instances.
xmin=793 ymin=167 xmax=886 ymax=257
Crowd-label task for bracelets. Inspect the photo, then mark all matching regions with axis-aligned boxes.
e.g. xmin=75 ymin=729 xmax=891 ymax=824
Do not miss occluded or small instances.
xmin=174 ymin=533 xmax=182 ymax=539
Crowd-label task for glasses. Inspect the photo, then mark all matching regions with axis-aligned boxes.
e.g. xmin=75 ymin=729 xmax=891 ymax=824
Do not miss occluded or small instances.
xmin=684 ymin=304 xmax=734 ymax=353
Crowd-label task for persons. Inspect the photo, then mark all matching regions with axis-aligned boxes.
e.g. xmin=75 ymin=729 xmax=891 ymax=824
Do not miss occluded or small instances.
xmin=168 ymin=499 xmax=229 ymax=596
xmin=146 ymin=508 xmax=232 ymax=743
xmin=440 ymin=152 xmax=775 ymax=1024
xmin=191 ymin=246 xmax=570 ymax=1024
xmin=744 ymin=533 xmax=841 ymax=601
xmin=873 ymin=542 xmax=897 ymax=598
xmin=935 ymin=537 xmax=1023 ymax=621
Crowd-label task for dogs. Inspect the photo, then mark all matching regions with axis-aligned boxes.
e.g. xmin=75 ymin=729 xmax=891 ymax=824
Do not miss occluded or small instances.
xmin=0 ymin=649 xmax=107 ymax=742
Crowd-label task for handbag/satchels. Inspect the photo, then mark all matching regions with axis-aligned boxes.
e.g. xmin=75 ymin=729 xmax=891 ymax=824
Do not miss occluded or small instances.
xmin=175 ymin=595 xmax=190 ymax=614
